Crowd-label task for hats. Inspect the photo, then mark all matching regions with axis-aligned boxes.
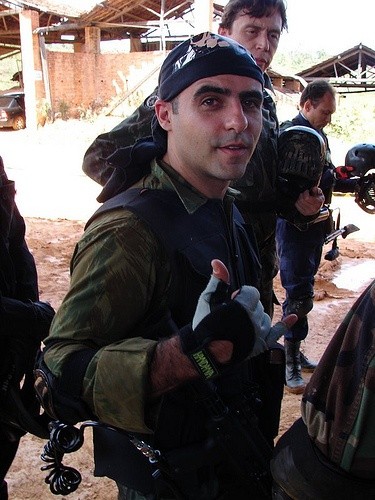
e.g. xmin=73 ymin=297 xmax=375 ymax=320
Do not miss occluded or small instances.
xmin=150 ymin=30 xmax=265 ymax=145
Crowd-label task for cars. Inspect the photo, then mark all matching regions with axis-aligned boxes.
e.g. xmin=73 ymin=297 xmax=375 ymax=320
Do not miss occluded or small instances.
xmin=0 ymin=87 xmax=26 ymax=131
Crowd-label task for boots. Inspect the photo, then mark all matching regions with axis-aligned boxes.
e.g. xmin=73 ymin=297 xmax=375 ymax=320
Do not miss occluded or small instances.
xmin=284 ymin=339 xmax=304 ymax=393
xmin=299 ymin=349 xmax=318 ymax=373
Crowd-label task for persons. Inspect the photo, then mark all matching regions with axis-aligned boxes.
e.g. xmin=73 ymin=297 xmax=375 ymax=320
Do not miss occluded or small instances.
xmin=32 ymin=31 xmax=299 ymax=500
xmin=0 ymin=155 xmax=54 ymax=500
xmin=267 ymin=279 xmax=375 ymax=500
xmin=277 ymin=79 xmax=336 ymax=395
xmin=81 ymin=0 xmax=326 ymax=321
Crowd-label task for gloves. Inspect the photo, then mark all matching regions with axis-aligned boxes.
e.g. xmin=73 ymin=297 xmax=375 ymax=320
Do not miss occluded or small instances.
xmin=336 ymin=163 xmax=354 ymax=181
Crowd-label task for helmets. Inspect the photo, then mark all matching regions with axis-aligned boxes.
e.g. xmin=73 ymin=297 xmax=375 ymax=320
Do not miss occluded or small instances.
xmin=276 ymin=125 xmax=326 ymax=188
xmin=344 ymin=143 xmax=375 ymax=177
